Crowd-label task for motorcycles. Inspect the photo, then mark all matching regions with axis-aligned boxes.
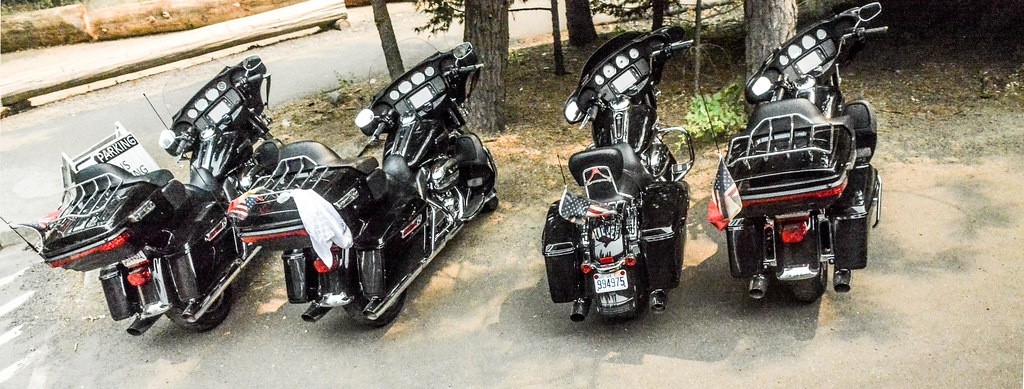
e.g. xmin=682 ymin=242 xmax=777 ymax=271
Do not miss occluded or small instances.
xmin=224 ymin=37 xmax=502 ymax=329
xmin=539 ymin=23 xmax=696 ymax=324
xmin=0 ymin=56 xmax=343 ymax=337
xmin=715 ymin=2 xmax=890 ymax=304
